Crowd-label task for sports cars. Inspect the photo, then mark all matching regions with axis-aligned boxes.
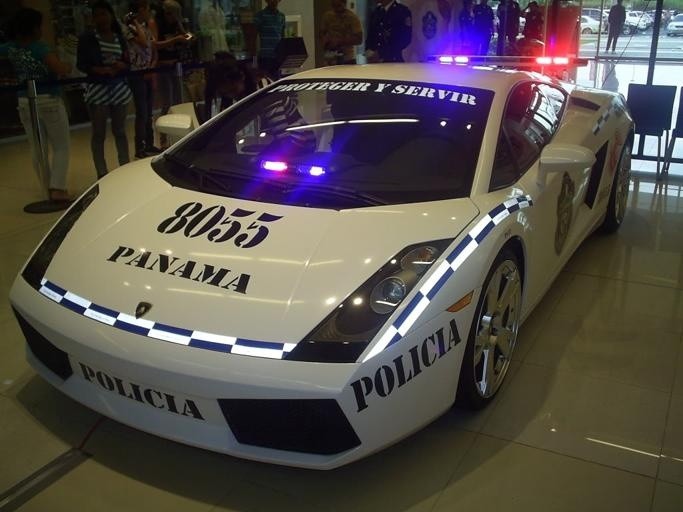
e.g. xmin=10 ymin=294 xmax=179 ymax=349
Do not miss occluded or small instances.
xmin=8 ymin=48 xmax=636 ymax=471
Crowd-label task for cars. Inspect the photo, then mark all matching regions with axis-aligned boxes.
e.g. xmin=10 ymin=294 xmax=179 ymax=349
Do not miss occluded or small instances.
xmin=577 ymin=9 xmax=682 ymax=37
xmin=491 ymin=6 xmax=526 ymax=34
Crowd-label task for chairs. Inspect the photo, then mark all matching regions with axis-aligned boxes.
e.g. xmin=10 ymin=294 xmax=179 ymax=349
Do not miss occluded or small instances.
xmin=622 ymin=81 xmax=676 ymax=183
xmin=658 ymin=85 xmax=682 ymax=179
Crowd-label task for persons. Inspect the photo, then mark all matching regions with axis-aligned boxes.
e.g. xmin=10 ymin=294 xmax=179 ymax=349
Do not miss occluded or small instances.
xmin=603 ymin=58 xmax=620 ymax=91
xmin=606 ymin=0 xmax=626 ymax=54
